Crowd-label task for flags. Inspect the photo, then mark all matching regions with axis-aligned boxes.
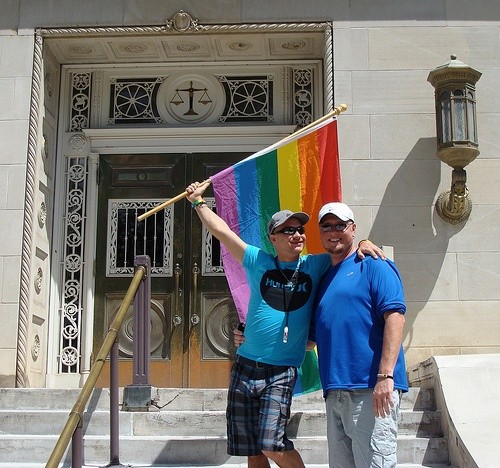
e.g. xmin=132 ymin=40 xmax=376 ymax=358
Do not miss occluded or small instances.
xmin=210 ymin=102 xmax=348 ymax=400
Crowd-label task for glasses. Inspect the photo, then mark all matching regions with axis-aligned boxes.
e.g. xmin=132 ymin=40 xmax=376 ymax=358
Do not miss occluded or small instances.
xmin=271 ymin=226 xmax=305 ymax=235
xmin=320 ymin=222 xmax=353 ymax=232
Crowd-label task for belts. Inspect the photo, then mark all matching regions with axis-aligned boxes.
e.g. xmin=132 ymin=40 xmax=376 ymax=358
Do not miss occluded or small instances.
xmin=237 ymin=354 xmax=273 ymax=370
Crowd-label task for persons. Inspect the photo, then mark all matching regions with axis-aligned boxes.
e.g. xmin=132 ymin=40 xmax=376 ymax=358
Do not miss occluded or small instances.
xmin=184 ymin=179 xmax=386 ymax=468
xmin=232 ymin=202 xmax=409 ymax=468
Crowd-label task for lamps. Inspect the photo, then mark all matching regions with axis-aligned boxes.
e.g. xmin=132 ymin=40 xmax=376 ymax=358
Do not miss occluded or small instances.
xmin=425 ymin=53 xmax=482 ymax=224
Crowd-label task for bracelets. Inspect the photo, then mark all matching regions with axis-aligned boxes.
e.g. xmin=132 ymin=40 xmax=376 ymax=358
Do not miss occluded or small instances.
xmin=191 ymin=198 xmax=208 ymax=210
xmin=376 ymin=374 xmax=393 ymax=380
xmin=358 ymin=238 xmax=372 ymax=247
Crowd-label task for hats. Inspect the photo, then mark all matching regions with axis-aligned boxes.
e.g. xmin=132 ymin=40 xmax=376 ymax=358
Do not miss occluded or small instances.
xmin=267 ymin=210 xmax=310 ymax=236
xmin=318 ymin=202 xmax=355 ymax=224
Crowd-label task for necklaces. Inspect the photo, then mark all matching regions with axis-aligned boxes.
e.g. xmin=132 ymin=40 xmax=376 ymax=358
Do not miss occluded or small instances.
xmin=275 ymin=257 xmax=301 ymax=289
